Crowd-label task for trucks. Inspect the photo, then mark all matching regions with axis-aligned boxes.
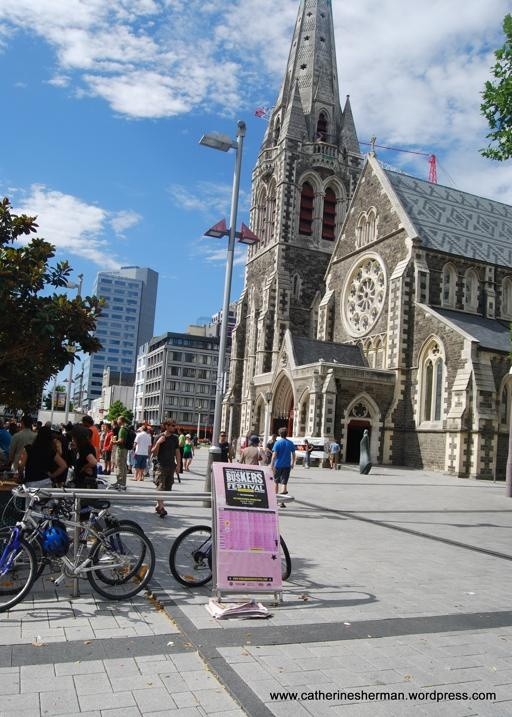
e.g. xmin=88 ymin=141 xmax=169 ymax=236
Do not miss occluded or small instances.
xmin=262 ymin=434 xmax=330 ymax=467
xmin=36 ymin=408 xmax=89 ymax=434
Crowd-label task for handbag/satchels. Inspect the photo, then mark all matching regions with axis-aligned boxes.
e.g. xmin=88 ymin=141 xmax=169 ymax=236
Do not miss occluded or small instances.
xmin=256 ymin=446 xmax=265 ymax=466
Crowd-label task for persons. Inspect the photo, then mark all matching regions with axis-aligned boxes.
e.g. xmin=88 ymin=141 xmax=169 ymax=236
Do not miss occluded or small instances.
xmin=268 ymin=427 xmax=296 ymax=495
xmin=329 ymin=441 xmax=340 ymax=469
xmin=218 ymin=432 xmax=277 ymax=467
xmin=303 ymin=440 xmax=314 ymax=469
xmin=0 ymin=416 xmax=199 ymax=561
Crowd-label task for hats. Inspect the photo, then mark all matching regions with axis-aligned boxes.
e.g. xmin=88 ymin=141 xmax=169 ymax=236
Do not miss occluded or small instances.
xmin=247 ymin=435 xmax=260 ymax=446
xmin=184 ymin=433 xmax=192 ymax=438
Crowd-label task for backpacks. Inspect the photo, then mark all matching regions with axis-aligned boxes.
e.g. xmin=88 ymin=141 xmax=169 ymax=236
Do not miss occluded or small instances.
xmin=121 ymin=427 xmax=136 ymax=450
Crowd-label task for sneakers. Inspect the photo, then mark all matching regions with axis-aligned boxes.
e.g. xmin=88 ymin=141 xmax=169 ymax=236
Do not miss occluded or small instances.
xmin=102 ymin=470 xmax=107 ymax=474
xmin=111 ymin=483 xmax=127 ymax=493
xmin=159 ymin=507 xmax=168 ymax=517
xmin=104 ymin=470 xmax=111 ymax=475
xmin=155 ymin=506 xmax=160 ymax=514
xmin=279 ymin=503 xmax=286 ymax=508
xmin=144 ymin=472 xmax=150 ymax=478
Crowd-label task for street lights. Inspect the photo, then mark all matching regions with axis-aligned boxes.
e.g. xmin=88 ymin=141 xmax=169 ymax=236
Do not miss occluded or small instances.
xmin=143 ymin=407 xmax=147 ymax=422
xmin=59 ymin=268 xmax=91 ymax=429
xmin=164 ymin=410 xmax=169 ymax=419
xmin=196 ymin=405 xmax=203 ymax=444
xmin=194 ymin=118 xmax=259 ymax=503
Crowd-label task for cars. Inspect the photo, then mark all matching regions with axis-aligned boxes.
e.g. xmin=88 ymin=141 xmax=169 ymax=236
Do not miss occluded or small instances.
xmin=198 ymin=437 xmax=210 ymax=444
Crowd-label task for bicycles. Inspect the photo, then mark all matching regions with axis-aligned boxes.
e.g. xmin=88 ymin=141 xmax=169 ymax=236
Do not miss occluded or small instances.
xmin=0 ymin=481 xmax=157 ymax=609
xmin=169 ymin=498 xmax=292 ymax=590
xmin=0 ymin=487 xmax=147 ymax=587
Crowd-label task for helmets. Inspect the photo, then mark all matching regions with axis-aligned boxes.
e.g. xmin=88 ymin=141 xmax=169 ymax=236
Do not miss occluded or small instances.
xmin=41 ymin=526 xmax=70 ymax=558
